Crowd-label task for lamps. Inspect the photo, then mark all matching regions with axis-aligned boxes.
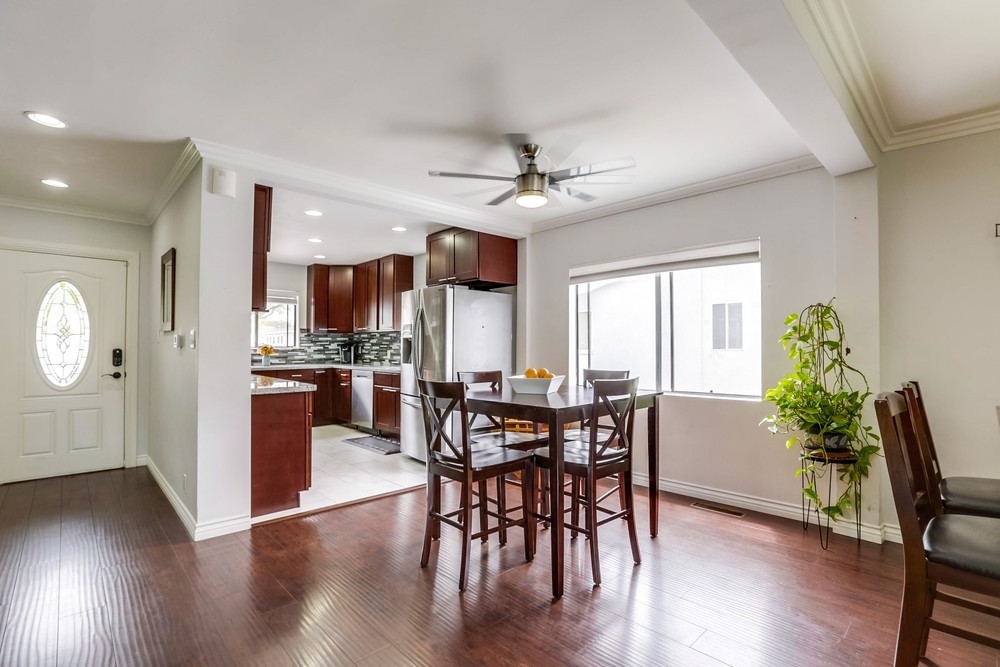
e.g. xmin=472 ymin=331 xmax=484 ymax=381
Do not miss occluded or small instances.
xmin=513 ymin=174 xmax=550 ymax=208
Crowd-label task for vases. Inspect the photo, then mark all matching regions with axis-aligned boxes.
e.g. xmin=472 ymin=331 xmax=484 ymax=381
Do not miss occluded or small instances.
xmin=262 ymin=355 xmax=270 ymax=367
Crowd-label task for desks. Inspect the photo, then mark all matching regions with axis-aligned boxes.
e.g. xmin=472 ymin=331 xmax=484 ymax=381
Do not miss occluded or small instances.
xmin=433 ymin=389 xmax=663 ymax=600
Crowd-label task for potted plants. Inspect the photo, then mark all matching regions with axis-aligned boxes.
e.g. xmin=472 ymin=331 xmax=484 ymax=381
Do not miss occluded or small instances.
xmin=759 ymin=295 xmax=884 ymax=523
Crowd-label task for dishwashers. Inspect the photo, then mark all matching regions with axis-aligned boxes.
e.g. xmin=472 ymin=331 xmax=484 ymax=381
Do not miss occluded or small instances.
xmin=351 ymin=369 xmax=374 ymax=429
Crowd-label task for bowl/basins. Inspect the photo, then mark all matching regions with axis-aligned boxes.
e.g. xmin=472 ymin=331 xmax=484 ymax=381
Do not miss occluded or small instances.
xmin=506 ymin=374 xmax=566 ymax=395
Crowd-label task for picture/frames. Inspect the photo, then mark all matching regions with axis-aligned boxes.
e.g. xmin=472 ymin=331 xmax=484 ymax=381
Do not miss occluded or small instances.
xmin=161 ymin=247 xmax=175 ymax=333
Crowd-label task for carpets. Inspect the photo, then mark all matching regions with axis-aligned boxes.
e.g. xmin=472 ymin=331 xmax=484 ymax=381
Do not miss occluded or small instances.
xmin=342 ymin=435 xmax=401 ymax=456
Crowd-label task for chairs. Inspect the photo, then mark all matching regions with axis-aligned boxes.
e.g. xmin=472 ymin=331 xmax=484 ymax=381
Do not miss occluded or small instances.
xmin=416 ymin=369 xmax=640 ymax=592
xmin=873 ymin=378 xmax=1000 ymax=667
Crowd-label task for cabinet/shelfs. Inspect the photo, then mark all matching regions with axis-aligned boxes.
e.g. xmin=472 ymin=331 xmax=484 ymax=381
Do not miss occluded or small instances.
xmin=251 ymin=368 xmax=401 ymax=516
xmin=306 ymin=254 xmax=414 ymax=336
xmin=425 ymin=227 xmax=518 ymax=291
xmin=251 ymin=182 xmax=273 ymax=313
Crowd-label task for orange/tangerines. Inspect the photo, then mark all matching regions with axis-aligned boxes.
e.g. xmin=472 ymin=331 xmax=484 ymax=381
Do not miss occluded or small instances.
xmin=525 ymin=368 xmax=554 ymax=378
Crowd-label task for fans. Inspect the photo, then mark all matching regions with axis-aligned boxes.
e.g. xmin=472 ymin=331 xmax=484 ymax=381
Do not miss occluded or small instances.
xmin=426 ymin=142 xmax=636 ymax=208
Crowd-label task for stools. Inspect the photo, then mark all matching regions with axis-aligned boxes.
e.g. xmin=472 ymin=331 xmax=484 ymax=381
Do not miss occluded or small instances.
xmin=799 ymin=450 xmax=863 ymax=551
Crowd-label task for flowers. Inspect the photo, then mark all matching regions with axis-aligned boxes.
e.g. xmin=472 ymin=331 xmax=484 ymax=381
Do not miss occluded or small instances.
xmin=260 ymin=344 xmax=276 ymax=356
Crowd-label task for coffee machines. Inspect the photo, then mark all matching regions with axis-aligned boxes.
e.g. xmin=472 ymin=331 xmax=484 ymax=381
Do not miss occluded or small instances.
xmin=339 ymin=340 xmax=358 ymax=364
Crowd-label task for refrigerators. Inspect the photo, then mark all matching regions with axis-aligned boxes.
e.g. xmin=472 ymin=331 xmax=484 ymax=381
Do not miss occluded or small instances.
xmin=400 ymin=284 xmax=513 ymax=462
